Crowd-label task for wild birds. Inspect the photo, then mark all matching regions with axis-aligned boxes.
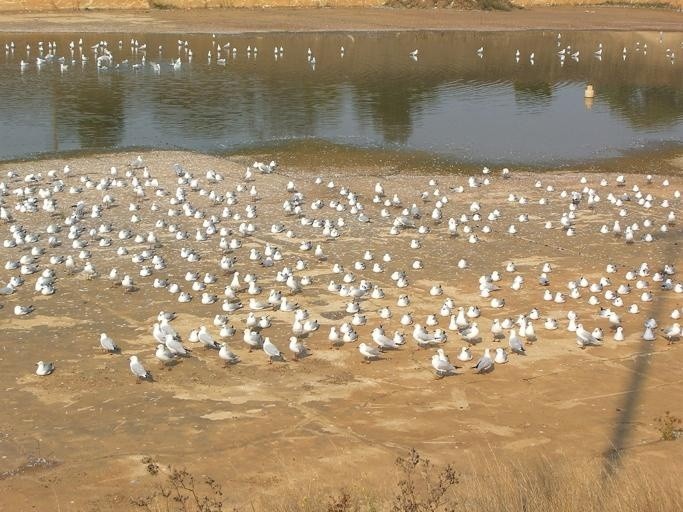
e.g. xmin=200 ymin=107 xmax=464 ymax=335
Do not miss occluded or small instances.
xmin=558 ymin=33 xmax=681 ymax=61
xmin=409 ymin=49 xmax=419 ymax=57
xmin=515 ymin=49 xmax=520 ymax=56
xmin=528 ymin=52 xmax=535 ymax=59
xmin=475 ymin=46 xmax=484 ymax=53
xmin=3 ymin=34 xmax=285 ymax=75
xmin=0 ymin=154 xmax=683 ymax=388
